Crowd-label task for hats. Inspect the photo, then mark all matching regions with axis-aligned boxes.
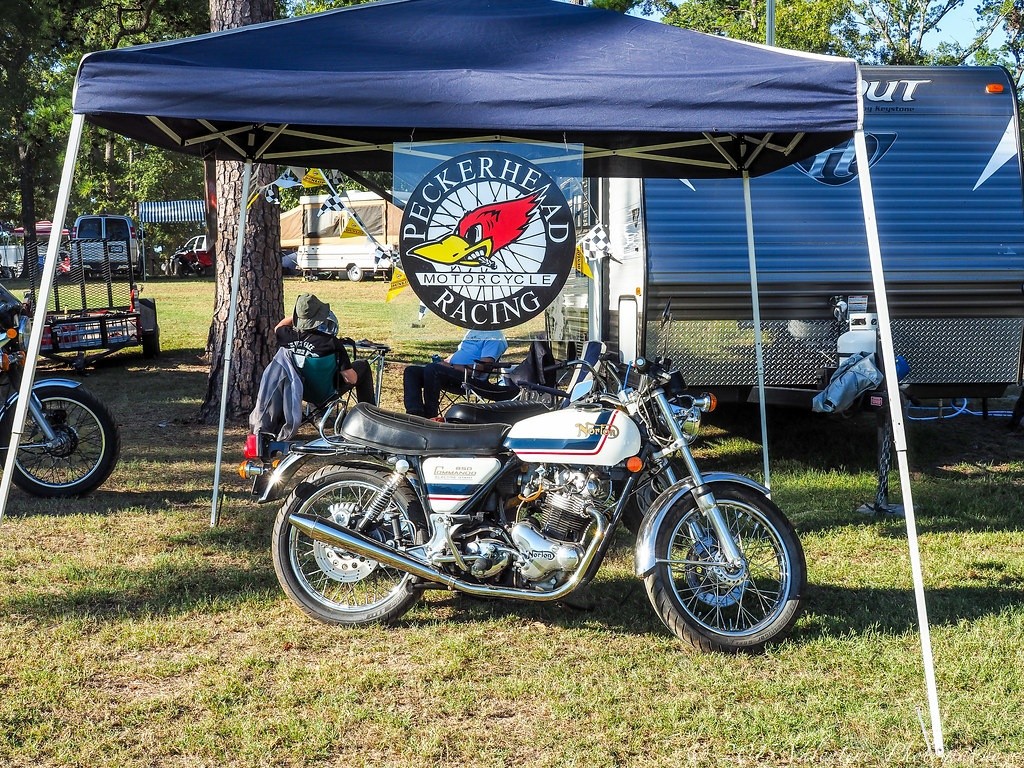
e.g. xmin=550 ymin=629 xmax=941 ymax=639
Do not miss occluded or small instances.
xmin=292 ymin=292 xmax=330 ymax=331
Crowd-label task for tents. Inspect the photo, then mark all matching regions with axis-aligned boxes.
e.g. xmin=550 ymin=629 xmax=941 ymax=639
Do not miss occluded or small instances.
xmin=139 ymin=201 xmax=205 ymax=282
xmin=14 ymin=221 xmax=70 ymax=265
xmin=0 ymin=0 xmax=944 ymax=757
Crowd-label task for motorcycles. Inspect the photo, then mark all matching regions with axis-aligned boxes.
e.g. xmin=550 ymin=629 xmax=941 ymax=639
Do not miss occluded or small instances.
xmin=0 ymin=300 xmax=121 ymax=499
xmin=239 ymin=296 xmax=807 ymax=655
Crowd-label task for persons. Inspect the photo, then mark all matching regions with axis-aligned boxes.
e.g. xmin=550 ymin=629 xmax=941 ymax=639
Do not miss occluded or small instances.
xmin=272 ymin=294 xmax=376 ymax=407
xmin=403 ymin=330 xmax=508 ymax=419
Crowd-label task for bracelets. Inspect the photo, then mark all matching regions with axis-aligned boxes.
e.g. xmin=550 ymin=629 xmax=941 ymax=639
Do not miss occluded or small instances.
xmin=450 ymin=364 xmax=453 ymax=368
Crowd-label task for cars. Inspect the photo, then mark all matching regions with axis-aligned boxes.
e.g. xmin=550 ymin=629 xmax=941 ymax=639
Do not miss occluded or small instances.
xmin=175 ymin=235 xmax=212 ymax=267
xmin=70 ymin=214 xmax=143 ymax=278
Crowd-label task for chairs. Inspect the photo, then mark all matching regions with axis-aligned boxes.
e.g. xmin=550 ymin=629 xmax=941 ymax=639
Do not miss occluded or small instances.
xmin=279 ymin=347 xmax=359 ymax=442
xmin=460 ymin=359 xmax=523 ymax=403
xmin=431 ymin=354 xmax=501 ymax=428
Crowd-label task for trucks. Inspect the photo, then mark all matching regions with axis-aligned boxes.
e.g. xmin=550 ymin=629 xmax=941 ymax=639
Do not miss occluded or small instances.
xmin=297 ymin=195 xmax=407 ymax=283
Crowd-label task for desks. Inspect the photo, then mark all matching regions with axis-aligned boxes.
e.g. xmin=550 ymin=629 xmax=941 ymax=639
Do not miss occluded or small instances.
xmin=344 ymin=340 xmax=392 ymax=409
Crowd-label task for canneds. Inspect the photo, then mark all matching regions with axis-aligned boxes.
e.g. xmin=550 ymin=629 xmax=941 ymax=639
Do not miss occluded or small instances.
xmin=432 ymin=354 xmax=442 ymax=363
